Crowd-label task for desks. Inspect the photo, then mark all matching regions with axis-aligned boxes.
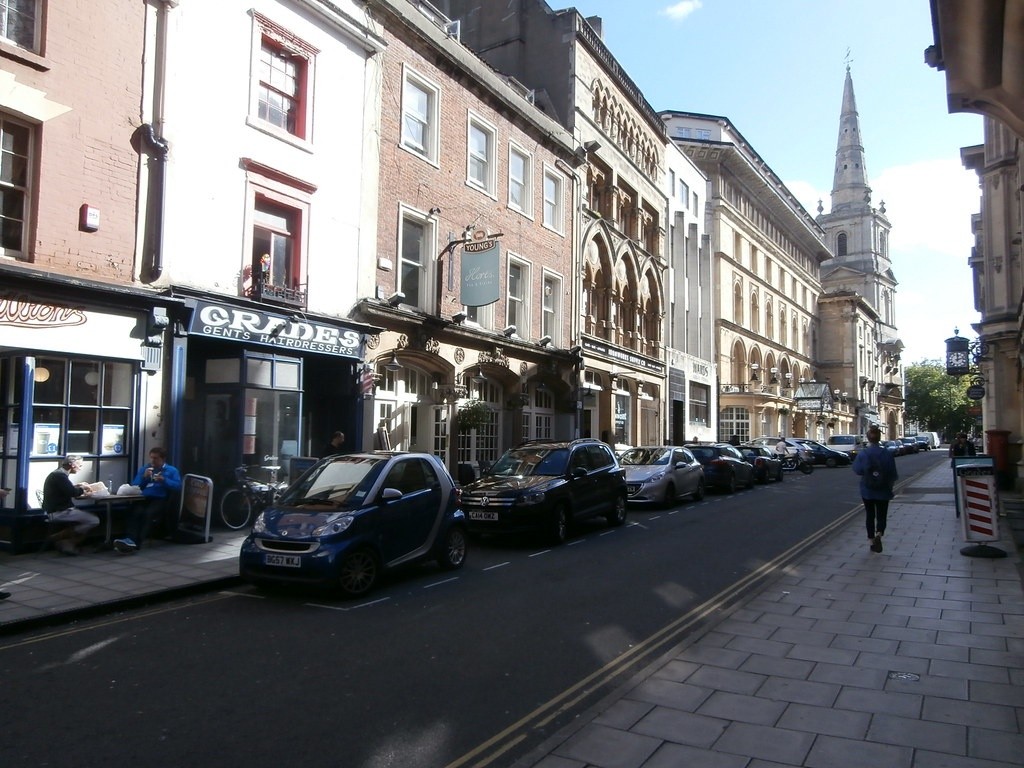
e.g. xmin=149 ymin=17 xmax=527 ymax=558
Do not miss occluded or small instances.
xmin=70 ymin=494 xmax=146 ymax=554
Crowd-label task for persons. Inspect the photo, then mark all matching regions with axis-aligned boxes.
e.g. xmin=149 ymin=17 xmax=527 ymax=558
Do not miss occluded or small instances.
xmin=0 ymin=488 xmax=12 ymax=600
xmin=323 ymin=432 xmax=344 ymax=458
xmin=114 ymin=447 xmax=183 ymax=555
xmin=953 ymin=434 xmax=977 ymax=457
xmin=852 ymin=425 xmax=898 ymax=553
xmin=948 ymin=433 xmax=961 ymax=457
xmin=42 ymin=455 xmax=100 ymax=557
xmin=728 ymin=435 xmax=740 ymax=447
xmin=776 ymin=437 xmax=790 ymax=465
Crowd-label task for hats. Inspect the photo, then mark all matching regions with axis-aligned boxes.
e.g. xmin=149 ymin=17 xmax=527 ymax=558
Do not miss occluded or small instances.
xmin=958 ymin=433 xmax=967 ymax=439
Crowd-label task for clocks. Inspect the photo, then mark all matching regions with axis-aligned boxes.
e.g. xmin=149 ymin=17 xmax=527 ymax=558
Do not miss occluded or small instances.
xmin=946 ymin=351 xmax=969 ymax=368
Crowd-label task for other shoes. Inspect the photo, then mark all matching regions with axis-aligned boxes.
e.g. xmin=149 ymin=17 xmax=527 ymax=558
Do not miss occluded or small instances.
xmin=0 ymin=592 xmax=10 ymax=602
xmin=58 ymin=543 xmax=71 ymax=554
xmin=871 ymin=544 xmax=875 ymax=551
xmin=873 ymin=536 xmax=882 ymax=552
xmin=113 ymin=538 xmax=136 ymax=552
xmin=50 ymin=535 xmax=64 ymax=551
xmin=114 ymin=547 xmax=134 ymax=555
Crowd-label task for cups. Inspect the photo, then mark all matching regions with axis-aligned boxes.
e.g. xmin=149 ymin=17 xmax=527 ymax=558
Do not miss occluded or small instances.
xmin=147 ymin=467 xmax=155 ymax=482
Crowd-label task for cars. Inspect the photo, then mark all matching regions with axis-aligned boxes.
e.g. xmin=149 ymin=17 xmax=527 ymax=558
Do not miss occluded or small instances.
xmin=896 ymin=440 xmax=905 ymax=457
xmin=898 ymin=437 xmax=919 ymax=455
xmin=617 ymin=446 xmax=706 ymax=508
xmin=684 ymin=443 xmax=756 ymax=495
xmin=851 ymin=442 xmax=885 ymax=465
xmin=803 ymin=440 xmax=850 ymax=467
xmin=740 ymin=437 xmax=816 ymax=471
xmin=882 ymin=440 xmax=897 ymax=457
xmin=734 ymin=446 xmax=783 ymax=485
xmin=916 ymin=436 xmax=932 ymax=451
xmin=239 ymin=453 xmax=467 ymax=597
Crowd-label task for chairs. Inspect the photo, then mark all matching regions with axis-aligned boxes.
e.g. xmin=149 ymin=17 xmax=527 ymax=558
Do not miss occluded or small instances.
xmin=36 ymin=489 xmax=80 ymax=556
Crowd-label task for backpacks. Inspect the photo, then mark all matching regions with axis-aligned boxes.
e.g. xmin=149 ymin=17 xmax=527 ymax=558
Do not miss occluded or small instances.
xmin=862 ymin=448 xmax=887 ymax=490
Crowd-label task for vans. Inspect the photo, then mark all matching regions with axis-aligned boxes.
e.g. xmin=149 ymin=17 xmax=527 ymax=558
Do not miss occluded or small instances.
xmin=919 ymin=432 xmax=940 ymax=449
xmin=826 ymin=435 xmax=862 ymax=460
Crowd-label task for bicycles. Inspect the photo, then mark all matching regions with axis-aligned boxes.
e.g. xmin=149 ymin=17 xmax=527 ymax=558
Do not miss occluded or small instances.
xmin=220 ymin=464 xmax=289 ymax=530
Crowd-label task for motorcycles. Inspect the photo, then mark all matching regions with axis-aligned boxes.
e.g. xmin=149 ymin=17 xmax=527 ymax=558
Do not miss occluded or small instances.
xmin=774 ymin=448 xmax=813 ymax=474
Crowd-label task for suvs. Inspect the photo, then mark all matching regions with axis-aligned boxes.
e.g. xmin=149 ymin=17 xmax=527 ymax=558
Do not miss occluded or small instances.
xmin=458 ymin=438 xmax=629 ymax=544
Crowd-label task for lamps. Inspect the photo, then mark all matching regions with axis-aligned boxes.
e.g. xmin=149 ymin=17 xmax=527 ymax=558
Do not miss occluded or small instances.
xmin=472 ymin=365 xmax=488 ymax=384
xmin=569 ymin=345 xmax=581 ymax=355
xmin=387 ymin=291 xmax=406 ymax=309
xmin=539 ymin=335 xmax=551 ymax=348
xmin=452 ymin=310 xmax=467 ymax=326
xmin=503 ymin=325 xmax=516 ymax=339
xmin=384 ymin=347 xmax=403 ymax=371
